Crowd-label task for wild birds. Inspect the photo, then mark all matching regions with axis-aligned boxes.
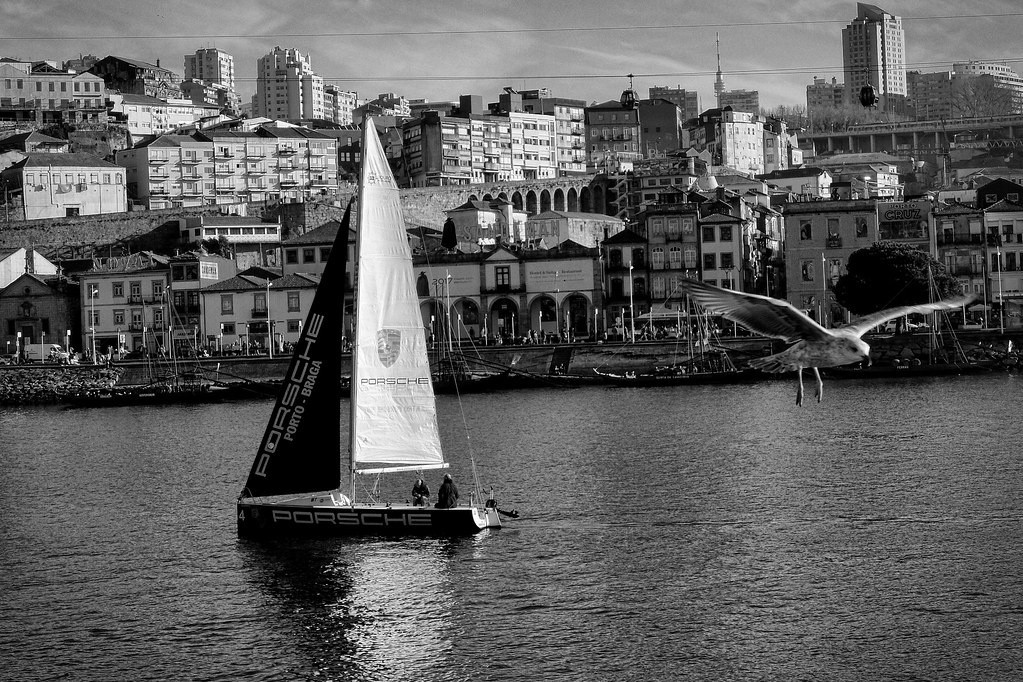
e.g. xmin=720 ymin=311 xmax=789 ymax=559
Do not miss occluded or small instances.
xmin=678 ymin=275 xmax=975 ymax=408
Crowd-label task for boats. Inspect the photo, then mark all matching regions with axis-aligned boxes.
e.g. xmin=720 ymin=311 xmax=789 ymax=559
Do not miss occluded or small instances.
xmin=481 ymin=368 xmax=581 ymax=390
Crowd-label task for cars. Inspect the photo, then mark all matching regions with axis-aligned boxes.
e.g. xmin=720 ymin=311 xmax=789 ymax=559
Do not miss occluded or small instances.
xmin=885 ymin=320 xmax=919 ymax=333
xmin=948 ymin=317 xmax=978 ymax=329
xmin=722 ymin=326 xmax=752 ymax=337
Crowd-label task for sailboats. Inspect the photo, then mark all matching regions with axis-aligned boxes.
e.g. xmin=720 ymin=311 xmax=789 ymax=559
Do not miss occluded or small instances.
xmin=54 ymin=288 xmax=229 ymax=407
xmin=236 ymin=115 xmax=502 ymax=532
xmin=804 ymin=251 xmax=1023 ymax=378
xmin=343 ymin=282 xmax=473 ymax=396
xmin=591 ymin=272 xmax=763 ymax=388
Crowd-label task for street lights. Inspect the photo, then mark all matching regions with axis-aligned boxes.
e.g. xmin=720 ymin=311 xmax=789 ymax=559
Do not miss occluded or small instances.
xmin=822 ymin=252 xmax=826 ymax=328
xmin=447 ymin=274 xmax=452 ymax=351
xmin=629 ymin=260 xmax=634 ymax=343
xmin=997 ymin=246 xmax=1003 ymax=334
xmin=266 ymin=282 xmax=273 ymax=360
xmin=92 ymin=290 xmax=99 ymax=365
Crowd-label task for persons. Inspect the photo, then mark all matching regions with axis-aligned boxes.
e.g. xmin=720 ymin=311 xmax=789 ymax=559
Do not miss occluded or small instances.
xmin=509 ymin=331 xmax=513 ymax=344
xmin=435 ymin=473 xmax=459 ymax=508
xmin=469 ymin=327 xmax=475 ymax=341
xmin=84 ymin=348 xmax=91 ymax=361
xmin=502 ymin=329 xmax=508 ymax=345
xmin=50 ymin=348 xmax=55 ymax=361
xmin=526 ymin=328 xmax=575 ymax=345
xmin=480 ymin=327 xmax=485 ymax=338
xmin=26 ymin=351 xmax=32 ymax=364
xmin=412 ymin=479 xmax=430 ymax=506
xmin=109 ymin=346 xmax=115 ymax=361
xmin=641 ymin=323 xmax=719 ymax=340
xmin=496 ymin=332 xmax=501 ymax=343
xmin=226 ymin=339 xmax=260 ymax=355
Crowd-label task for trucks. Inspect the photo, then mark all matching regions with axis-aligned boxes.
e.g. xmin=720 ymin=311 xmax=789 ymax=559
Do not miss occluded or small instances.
xmin=25 ymin=344 xmax=65 ymax=364
